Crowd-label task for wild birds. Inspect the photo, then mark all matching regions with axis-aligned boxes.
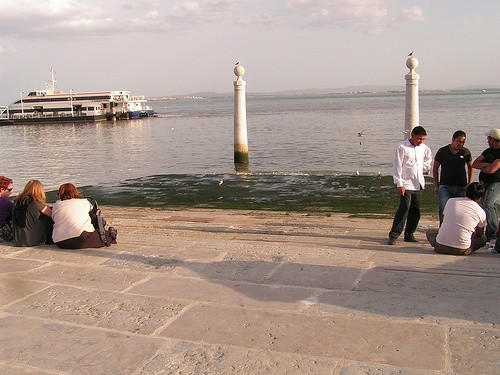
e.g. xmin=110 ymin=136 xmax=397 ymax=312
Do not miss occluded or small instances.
xmin=357 ymin=129 xmax=366 ymax=136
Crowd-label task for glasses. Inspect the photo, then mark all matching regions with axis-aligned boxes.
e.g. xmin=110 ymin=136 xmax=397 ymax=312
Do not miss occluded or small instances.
xmin=6 ymin=188 xmax=13 ymax=191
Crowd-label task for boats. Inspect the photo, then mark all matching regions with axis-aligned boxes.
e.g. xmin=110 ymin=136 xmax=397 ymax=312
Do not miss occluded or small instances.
xmin=0 ymin=65 xmax=160 ymax=125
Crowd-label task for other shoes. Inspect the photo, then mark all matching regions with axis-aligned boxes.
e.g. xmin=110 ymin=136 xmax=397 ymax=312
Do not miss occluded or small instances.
xmin=388 ymin=236 xmax=397 ymax=244
xmin=403 ymin=232 xmax=418 ymax=242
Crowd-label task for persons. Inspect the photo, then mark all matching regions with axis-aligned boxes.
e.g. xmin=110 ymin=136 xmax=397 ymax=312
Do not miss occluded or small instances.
xmin=0 ymin=176 xmax=20 ymax=243
xmin=471 ymin=128 xmax=500 ymax=250
xmin=51 ymin=183 xmax=116 ymax=249
xmin=427 ymin=182 xmax=487 ymax=256
xmin=433 ymin=130 xmax=473 ymax=230
xmin=388 ymin=125 xmax=432 ymax=245
xmin=12 ymin=178 xmax=54 ymax=247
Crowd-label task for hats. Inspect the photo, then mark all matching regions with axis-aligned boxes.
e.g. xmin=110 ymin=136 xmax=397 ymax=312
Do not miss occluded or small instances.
xmin=485 ymin=128 xmax=500 ymax=140
